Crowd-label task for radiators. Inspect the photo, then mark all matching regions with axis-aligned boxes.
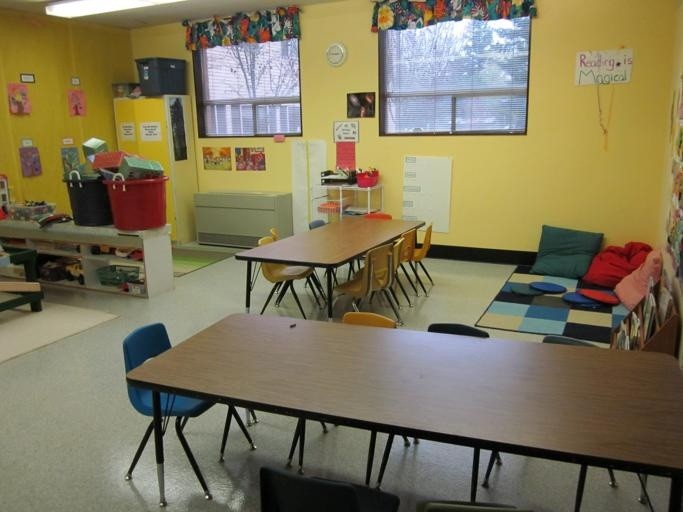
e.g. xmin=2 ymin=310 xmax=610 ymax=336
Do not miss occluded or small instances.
xmin=192 ymin=191 xmax=292 ymax=248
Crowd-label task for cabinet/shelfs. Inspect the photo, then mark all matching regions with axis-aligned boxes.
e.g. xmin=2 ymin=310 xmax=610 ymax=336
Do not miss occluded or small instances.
xmin=308 ymin=182 xmax=384 ymax=229
xmin=111 ymin=94 xmax=196 ymax=247
xmin=610 ymin=284 xmax=679 ymax=360
xmin=0 ymin=220 xmax=173 ymax=296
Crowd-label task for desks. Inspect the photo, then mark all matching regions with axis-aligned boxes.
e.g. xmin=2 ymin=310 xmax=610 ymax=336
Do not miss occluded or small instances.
xmin=125 ymin=314 xmax=683 ymax=509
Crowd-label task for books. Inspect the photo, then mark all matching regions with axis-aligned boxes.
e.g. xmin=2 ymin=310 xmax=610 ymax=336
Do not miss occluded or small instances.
xmin=608 ymin=275 xmax=679 ymax=351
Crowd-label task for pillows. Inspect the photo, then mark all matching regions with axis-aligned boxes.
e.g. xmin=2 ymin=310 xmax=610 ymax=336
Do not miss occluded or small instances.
xmin=613 ymin=247 xmax=666 ymax=312
xmin=529 ymin=223 xmax=606 ymax=276
xmin=585 ymin=240 xmax=649 ymax=290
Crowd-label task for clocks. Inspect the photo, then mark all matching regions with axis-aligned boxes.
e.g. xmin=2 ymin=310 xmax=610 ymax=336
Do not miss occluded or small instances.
xmin=323 ymin=42 xmax=347 ymax=68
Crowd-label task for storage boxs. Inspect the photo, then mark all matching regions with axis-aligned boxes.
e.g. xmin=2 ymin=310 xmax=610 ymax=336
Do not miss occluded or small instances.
xmin=133 ymin=55 xmax=187 ymax=95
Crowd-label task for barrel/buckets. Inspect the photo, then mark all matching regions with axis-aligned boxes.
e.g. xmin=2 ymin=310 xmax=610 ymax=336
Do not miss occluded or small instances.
xmin=101 ymin=173 xmax=169 ymax=230
xmin=62 ymin=170 xmax=113 ymax=227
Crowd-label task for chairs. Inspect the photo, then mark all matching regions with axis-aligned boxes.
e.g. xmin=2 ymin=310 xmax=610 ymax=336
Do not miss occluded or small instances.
xmin=122 ymin=324 xmax=258 ymax=495
xmin=290 ymin=313 xmax=399 ymax=483
xmin=258 ymin=466 xmax=402 ymax=511
xmin=0 ymin=245 xmax=46 ymax=318
xmin=474 ymin=334 xmax=603 ymax=509
xmin=235 ymin=211 xmax=434 ymax=322
xmin=373 ymin=322 xmax=503 ymax=503
xmin=417 ymin=495 xmax=522 ymax=512
xmin=217 ymin=402 xmax=329 ymax=473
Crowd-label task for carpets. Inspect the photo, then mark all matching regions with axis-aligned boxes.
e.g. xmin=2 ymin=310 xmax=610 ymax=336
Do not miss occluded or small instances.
xmin=0 ymin=296 xmax=120 ymax=365
xmin=474 ymin=255 xmax=631 ymax=343
xmin=171 ymin=239 xmax=246 ymax=282
